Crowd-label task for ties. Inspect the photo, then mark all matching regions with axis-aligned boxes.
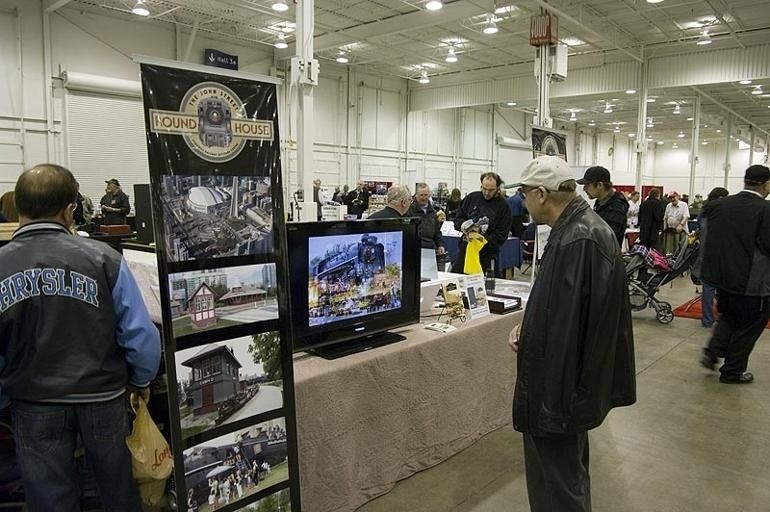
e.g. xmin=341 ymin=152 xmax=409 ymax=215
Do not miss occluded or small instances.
xmin=287 ymin=217 xmax=422 ymax=360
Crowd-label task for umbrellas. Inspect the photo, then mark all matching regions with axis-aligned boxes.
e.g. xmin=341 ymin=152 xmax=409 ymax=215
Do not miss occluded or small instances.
xmin=720 ymin=373 xmax=753 ymax=383
xmin=700 ymin=355 xmax=716 ymax=369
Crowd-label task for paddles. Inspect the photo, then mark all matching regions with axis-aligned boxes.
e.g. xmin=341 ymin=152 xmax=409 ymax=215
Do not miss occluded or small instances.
xmin=672 ymin=293 xmax=770 ymax=329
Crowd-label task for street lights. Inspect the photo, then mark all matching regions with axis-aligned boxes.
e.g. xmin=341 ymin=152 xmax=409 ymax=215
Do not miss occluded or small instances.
xmin=463 ymin=234 xmax=488 ymax=275
xmin=126 ymin=396 xmax=172 ymax=480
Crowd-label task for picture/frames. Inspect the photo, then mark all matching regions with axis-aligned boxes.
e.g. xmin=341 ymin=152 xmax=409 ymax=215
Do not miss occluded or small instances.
xmin=291 ymin=299 xmax=532 ymax=510
xmin=441 ymin=231 xmax=519 ymax=279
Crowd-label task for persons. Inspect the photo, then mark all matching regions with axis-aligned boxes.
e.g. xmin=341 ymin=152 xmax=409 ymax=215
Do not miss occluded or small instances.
xmin=627 ymin=188 xmax=692 ymax=261
xmin=575 ymin=168 xmax=630 ymax=255
xmin=72 ymin=175 xmax=132 ymax=228
xmin=699 ymin=188 xmax=731 ymax=326
xmin=503 ymin=154 xmax=635 ymax=511
xmin=453 ymin=171 xmax=512 ymax=276
xmin=0 ymin=163 xmax=163 ymax=509
xmin=701 ymin=167 xmax=768 ymax=385
xmin=312 ymin=177 xmax=464 ymax=271
xmin=507 ymin=186 xmax=530 ymax=238
xmin=187 ymin=444 xmax=271 ymax=510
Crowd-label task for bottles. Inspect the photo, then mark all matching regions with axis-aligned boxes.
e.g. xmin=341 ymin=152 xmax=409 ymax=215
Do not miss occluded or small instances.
xmin=744 ymin=165 xmax=770 ymax=185
xmin=576 ymin=167 xmax=610 ymax=184
xmin=504 ymin=156 xmax=574 ymax=191
xmin=105 ymin=179 xmax=119 ymax=186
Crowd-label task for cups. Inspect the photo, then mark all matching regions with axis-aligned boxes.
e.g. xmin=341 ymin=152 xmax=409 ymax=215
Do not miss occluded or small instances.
xmin=517 ymin=187 xmax=550 ymax=199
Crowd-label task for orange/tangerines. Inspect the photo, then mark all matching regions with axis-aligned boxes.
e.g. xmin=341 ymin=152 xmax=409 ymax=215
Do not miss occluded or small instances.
xmin=612 ymin=236 xmax=699 ymax=325
